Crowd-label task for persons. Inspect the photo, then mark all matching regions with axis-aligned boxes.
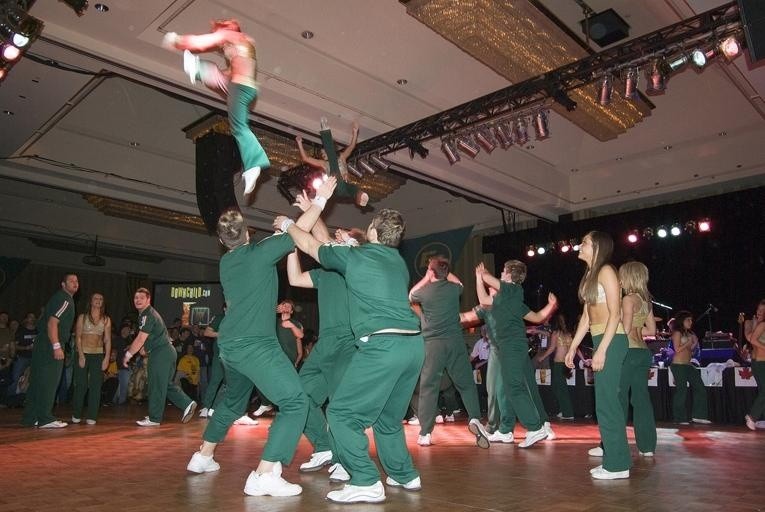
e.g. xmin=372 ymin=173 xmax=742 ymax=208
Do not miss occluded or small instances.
xmin=745 ymin=300 xmax=765 ymax=428
xmin=71 ymin=291 xmax=111 ymax=424
xmin=122 ymin=287 xmax=198 ymax=427
xmin=20 ymin=273 xmax=79 ymax=429
xmin=293 ymin=115 xmax=370 ymax=208
xmin=184 ymin=174 xmax=337 ymax=497
xmin=586 ymin=260 xmax=658 ymax=458
xmin=664 ymin=318 xmax=702 ymax=367
xmin=161 ymin=17 xmax=272 ymax=199
xmin=564 ymin=229 xmax=635 ymax=480
xmin=270 ymin=183 xmax=427 ymax=504
xmin=744 ymin=320 xmax=765 ymax=431
xmin=271 ymin=187 xmax=372 ymax=483
xmin=538 ymin=314 xmax=591 ymax=420
xmin=668 ymin=311 xmax=713 ymax=426
xmin=169 ymin=223 xmax=557 ymax=456
xmin=1 ymin=309 xmax=147 ymax=414
xmin=53 ymin=336 xmax=74 ymax=408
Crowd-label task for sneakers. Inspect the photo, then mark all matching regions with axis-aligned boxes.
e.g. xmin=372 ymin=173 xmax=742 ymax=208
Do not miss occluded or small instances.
xmin=692 ymin=418 xmax=712 ymax=424
xmin=244 ymin=450 xmax=422 ymax=503
xmin=183 ymin=49 xmax=200 ymax=84
xmin=745 ymin=415 xmax=755 ymax=431
xmin=673 ymin=422 xmax=690 ymax=425
xmin=408 ymin=399 xmax=575 ymax=449
xmin=242 ymin=166 xmax=261 ymax=196
xmin=187 ymin=451 xmax=221 ymax=473
xmin=588 ymin=446 xmax=654 ymax=480
xmin=756 ymin=421 xmax=765 ymax=428
xmin=35 ymin=400 xmax=273 ymax=429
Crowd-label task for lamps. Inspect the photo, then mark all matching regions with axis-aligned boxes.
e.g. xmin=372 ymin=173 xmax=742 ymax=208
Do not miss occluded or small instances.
xmin=628 ymin=216 xmax=712 ymax=244
xmin=0 ymin=2 xmax=46 ymax=82
xmin=524 ymin=236 xmax=582 ymax=257
xmin=346 ymin=154 xmax=391 ymax=178
xmin=600 ymin=65 xmax=665 ymax=107
xmin=440 ymin=101 xmax=550 ymax=165
xmin=663 ymin=33 xmax=741 ymax=73
